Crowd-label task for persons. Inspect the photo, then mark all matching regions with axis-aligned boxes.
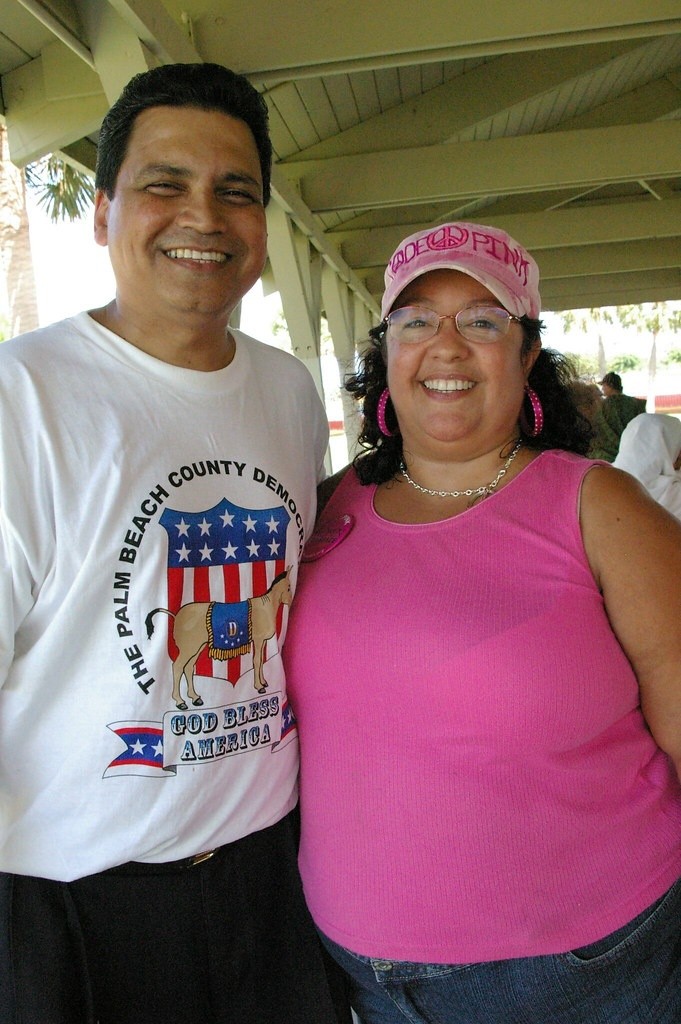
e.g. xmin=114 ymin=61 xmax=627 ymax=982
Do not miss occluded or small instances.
xmin=598 ymin=370 xmax=648 ymax=441
xmin=288 ymin=223 xmax=679 ymax=1024
xmin=561 ymin=381 xmax=620 ymax=463
xmin=0 ymin=67 xmax=328 ymax=1024
xmin=608 ymin=412 xmax=680 ymax=522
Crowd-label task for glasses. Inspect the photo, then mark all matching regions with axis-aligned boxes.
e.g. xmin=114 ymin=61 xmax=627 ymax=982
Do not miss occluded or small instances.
xmin=385 ymin=306 xmax=520 ymax=344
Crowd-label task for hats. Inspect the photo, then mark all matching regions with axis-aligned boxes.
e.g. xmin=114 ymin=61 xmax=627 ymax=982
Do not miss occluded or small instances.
xmin=598 ymin=372 xmax=621 ymax=387
xmin=380 ymin=222 xmax=541 ymax=323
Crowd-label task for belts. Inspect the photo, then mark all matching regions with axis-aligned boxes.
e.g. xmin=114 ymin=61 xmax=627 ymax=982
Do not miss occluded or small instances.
xmin=97 ymin=848 xmax=219 ymax=875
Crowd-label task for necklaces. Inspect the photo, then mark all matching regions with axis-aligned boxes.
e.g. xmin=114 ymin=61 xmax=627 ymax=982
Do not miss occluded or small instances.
xmin=401 ymin=437 xmax=524 ymax=497
